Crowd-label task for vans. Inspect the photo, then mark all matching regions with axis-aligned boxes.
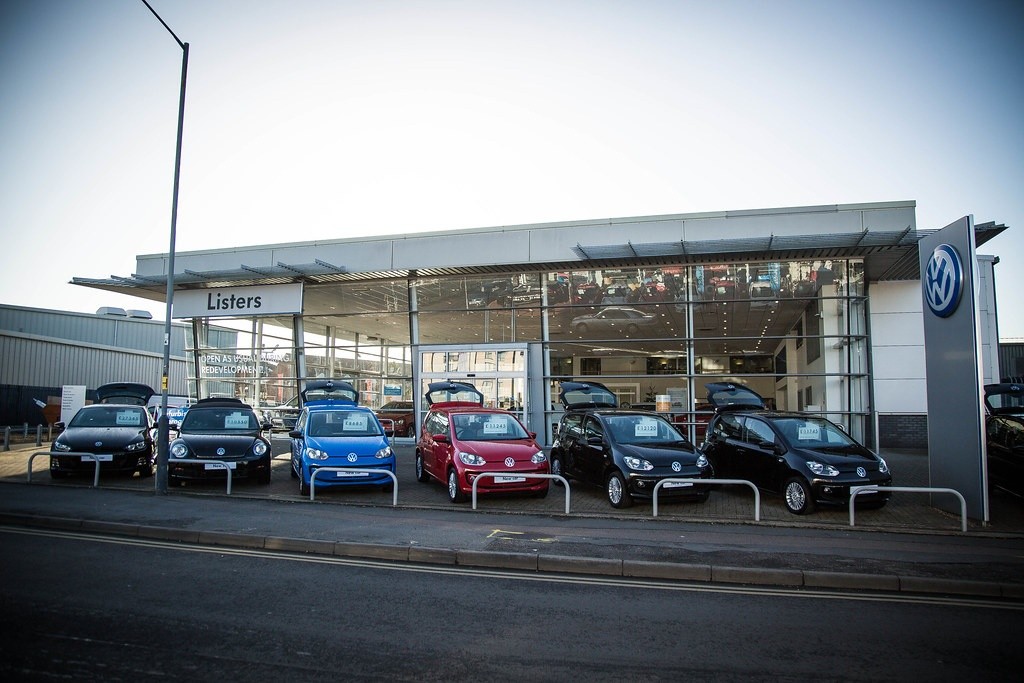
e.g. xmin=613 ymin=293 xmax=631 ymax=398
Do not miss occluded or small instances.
xmin=550 ymin=401 xmax=565 ymax=435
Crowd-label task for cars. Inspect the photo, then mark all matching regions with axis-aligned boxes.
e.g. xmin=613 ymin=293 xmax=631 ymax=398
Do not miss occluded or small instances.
xmin=698 ymin=381 xmax=893 ymax=516
xmin=550 ymin=380 xmax=713 ymax=509
xmin=372 ymin=399 xmax=429 ymax=439
xmin=50 ymin=381 xmax=158 ymax=480
xmin=415 ymin=381 xmax=550 ymax=504
xmin=570 ymin=304 xmax=659 ymax=336
xmin=668 ymin=403 xmax=714 ymax=436
xmin=168 ymin=397 xmax=272 ymax=488
xmin=146 ymin=394 xmax=303 ymax=433
xmin=468 ymin=255 xmax=821 ymax=315
xmin=985 ymin=383 xmax=1024 ymax=500
xmin=289 ymin=380 xmax=398 ymax=496
